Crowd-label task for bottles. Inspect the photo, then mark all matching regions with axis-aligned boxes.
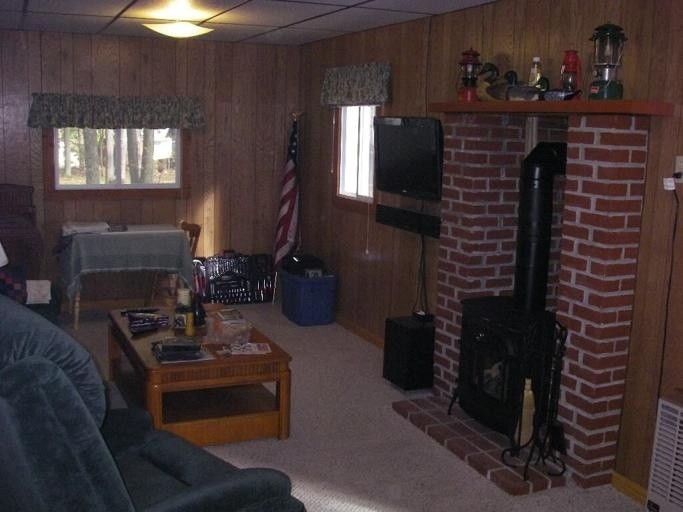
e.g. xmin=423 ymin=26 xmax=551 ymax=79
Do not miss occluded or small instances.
xmin=173 ymin=288 xmax=193 ymax=332
xmin=126 ymin=311 xmax=169 ymax=333
xmin=189 ymin=259 xmax=205 ymax=328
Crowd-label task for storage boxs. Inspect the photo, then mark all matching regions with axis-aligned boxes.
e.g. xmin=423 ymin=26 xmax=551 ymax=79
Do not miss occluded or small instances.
xmin=280 ymin=268 xmax=336 ymax=327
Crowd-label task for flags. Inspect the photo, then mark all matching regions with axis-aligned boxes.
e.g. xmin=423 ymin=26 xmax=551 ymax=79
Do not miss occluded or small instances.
xmin=268 ymin=111 xmax=300 ymax=270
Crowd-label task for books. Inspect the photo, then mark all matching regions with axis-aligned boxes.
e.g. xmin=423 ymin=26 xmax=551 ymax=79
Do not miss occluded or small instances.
xmin=215 ymin=308 xmax=242 ymax=323
xmin=154 ymin=344 xmax=203 ymax=362
xmin=160 ymin=337 xmax=202 ymax=353
xmin=229 ymin=341 xmax=272 ymax=356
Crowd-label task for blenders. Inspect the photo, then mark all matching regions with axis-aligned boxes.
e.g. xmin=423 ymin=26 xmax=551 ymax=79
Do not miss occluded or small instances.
xmin=587 ymin=24 xmax=628 ymax=101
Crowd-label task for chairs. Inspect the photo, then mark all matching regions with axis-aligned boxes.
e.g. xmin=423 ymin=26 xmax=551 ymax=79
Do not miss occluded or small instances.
xmin=0 ymin=184 xmax=44 ymax=302
xmin=0 ymin=294 xmax=306 ymax=512
xmin=144 ymin=218 xmax=200 ymax=306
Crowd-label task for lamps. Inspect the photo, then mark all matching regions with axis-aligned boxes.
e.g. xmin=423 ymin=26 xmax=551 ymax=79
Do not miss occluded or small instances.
xmin=142 ymin=19 xmax=214 ymax=38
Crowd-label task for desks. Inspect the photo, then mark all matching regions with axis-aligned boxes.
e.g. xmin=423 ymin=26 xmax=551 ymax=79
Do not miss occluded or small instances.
xmin=53 ymin=224 xmax=196 ymax=331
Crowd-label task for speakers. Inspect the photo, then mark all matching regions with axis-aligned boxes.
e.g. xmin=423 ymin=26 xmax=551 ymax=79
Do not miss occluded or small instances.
xmin=382 ymin=314 xmax=435 ymax=390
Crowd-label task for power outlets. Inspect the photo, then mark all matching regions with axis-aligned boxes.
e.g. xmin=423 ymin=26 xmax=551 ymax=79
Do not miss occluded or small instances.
xmin=675 ymin=155 xmax=683 ymax=183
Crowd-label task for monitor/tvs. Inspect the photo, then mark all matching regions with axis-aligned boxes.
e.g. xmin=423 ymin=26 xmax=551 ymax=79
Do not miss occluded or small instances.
xmin=373 ymin=115 xmax=443 ymax=201
